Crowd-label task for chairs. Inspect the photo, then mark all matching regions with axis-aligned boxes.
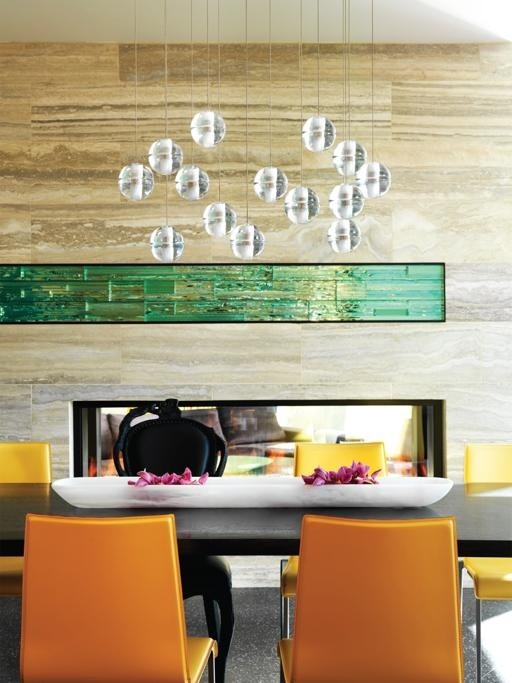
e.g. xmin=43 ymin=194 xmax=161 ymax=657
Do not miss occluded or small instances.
xmin=113 ymin=399 xmax=234 ymax=682
xmin=19 ymin=513 xmax=218 ymax=682
xmin=279 ymin=442 xmax=387 ymax=638
xmin=2 ymin=443 xmax=51 ymax=597
xmin=458 ymin=442 xmax=510 ymax=682
xmin=278 ymin=515 xmax=463 ymax=682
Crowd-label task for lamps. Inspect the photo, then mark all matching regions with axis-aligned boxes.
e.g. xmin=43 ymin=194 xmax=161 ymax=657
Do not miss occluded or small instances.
xmin=112 ymin=36 xmax=391 ymax=257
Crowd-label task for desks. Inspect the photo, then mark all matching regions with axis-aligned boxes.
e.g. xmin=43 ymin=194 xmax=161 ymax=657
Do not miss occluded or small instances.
xmin=1 ymin=482 xmax=512 ymax=557
